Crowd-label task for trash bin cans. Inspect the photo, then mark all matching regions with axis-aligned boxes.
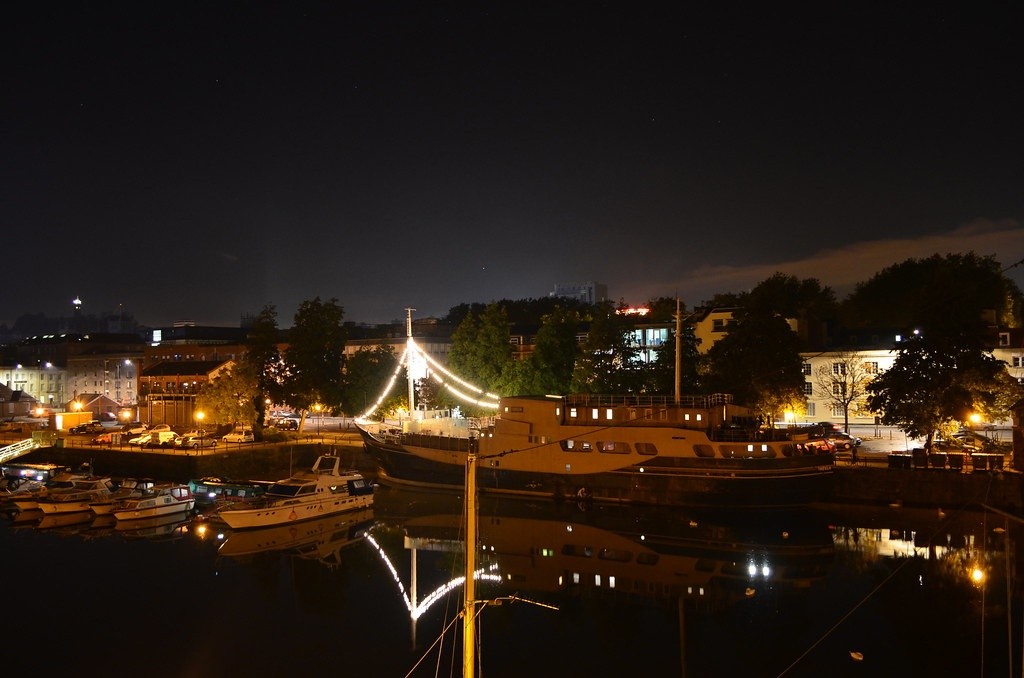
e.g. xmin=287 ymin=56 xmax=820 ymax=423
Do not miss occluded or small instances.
xmin=887 ymin=453 xmax=912 ymax=470
xmin=948 ymin=454 xmax=965 ymax=470
xmin=989 ymin=455 xmax=1005 ymax=471
xmin=931 ymin=452 xmax=948 ymax=469
xmin=971 ymin=453 xmax=988 ymax=471
xmin=913 ymin=449 xmax=929 ymax=469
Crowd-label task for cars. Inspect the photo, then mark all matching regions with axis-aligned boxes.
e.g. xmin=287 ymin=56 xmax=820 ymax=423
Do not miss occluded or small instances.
xmin=91 ymin=434 xmax=113 ymax=444
xmin=811 ymin=421 xmax=841 ymax=434
xmin=182 ymin=436 xmax=217 ymax=450
xmin=142 ymin=423 xmax=171 ymax=435
xmin=928 ymin=430 xmax=990 ymax=453
xmin=222 ymin=429 xmax=254 ymax=444
xmin=827 ymin=431 xmax=862 ymax=450
xmin=273 ymin=411 xmax=290 ymax=419
xmin=128 ymin=435 xmax=151 ymax=447
xmin=169 ymin=437 xmax=185 ymax=448
xmin=69 ymin=419 xmax=148 ymax=437
xmin=182 ymin=429 xmax=206 ymax=437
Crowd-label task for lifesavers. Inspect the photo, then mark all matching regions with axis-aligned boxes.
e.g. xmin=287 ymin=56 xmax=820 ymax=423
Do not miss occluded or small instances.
xmin=289 ymin=527 xmax=297 ymax=535
xmin=289 ymin=512 xmax=297 ymax=520
xmin=40 ymin=486 xmax=48 ymax=493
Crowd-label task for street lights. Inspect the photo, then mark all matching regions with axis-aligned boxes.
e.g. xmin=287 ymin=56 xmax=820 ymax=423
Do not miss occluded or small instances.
xmin=316 ymin=405 xmax=320 ymax=435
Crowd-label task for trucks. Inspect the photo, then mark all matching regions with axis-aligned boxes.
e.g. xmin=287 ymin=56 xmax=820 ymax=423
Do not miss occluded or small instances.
xmin=151 ymin=431 xmax=180 ymax=445
xmin=277 ymin=420 xmax=298 ymax=431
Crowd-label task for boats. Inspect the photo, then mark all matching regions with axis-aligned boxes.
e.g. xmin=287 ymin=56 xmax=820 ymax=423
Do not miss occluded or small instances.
xmin=114 ymin=511 xmax=192 ymax=546
xmin=190 ymin=477 xmax=276 ymax=505
xmin=0 ymin=462 xmax=71 ymax=508
xmin=218 ymin=454 xmax=373 ymax=531
xmin=7 ymin=512 xmax=113 ymax=542
xmin=36 ymin=478 xmax=113 ymax=515
xmin=216 ymin=509 xmax=374 ymax=570
xmin=9 ymin=474 xmax=91 ymax=512
xmin=88 ymin=477 xmax=157 ymax=516
xmin=108 ymin=483 xmax=195 ymax=521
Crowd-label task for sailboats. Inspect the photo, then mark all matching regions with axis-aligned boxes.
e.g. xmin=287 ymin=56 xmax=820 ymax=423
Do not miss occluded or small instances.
xmin=370 ymin=486 xmax=851 ymax=613
xmin=350 ymin=308 xmax=837 ymax=516
